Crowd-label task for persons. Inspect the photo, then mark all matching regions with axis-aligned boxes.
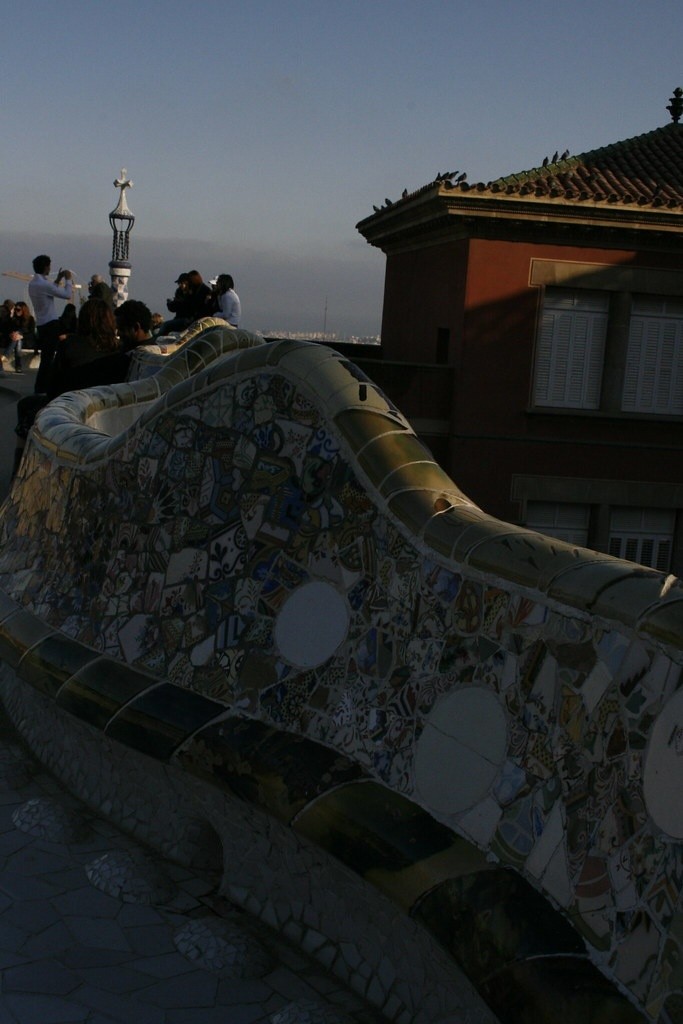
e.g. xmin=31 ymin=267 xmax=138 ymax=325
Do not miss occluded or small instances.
xmin=157 ymin=270 xmax=238 ymax=338
xmin=0 ymin=299 xmax=41 ymax=372
xmin=10 ymin=274 xmax=164 ymax=482
xmin=28 ymin=254 xmax=74 ymax=394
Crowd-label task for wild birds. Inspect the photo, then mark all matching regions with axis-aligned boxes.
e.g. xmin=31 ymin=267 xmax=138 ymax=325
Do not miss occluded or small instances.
xmin=372 ymin=188 xmax=408 ymax=212
xmin=542 ymin=148 xmax=570 ymax=166
xmin=435 ymin=170 xmax=467 ymax=184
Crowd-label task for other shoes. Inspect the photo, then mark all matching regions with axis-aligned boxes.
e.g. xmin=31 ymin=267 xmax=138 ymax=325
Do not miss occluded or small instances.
xmin=16 ymin=367 xmax=22 ymax=372
xmin=36 ymin=393 xmax=47 ymax=396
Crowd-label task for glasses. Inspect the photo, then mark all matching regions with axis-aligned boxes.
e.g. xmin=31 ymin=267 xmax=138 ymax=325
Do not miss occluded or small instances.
xmin=14 ymin=308 xmax=22 ymax=312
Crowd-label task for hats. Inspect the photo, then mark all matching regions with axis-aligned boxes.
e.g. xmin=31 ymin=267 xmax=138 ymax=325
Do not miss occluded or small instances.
xmin=175 ymin=274 xmax=189 ymax=282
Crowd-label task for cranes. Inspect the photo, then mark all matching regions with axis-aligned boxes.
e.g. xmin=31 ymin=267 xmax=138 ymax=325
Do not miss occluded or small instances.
xmin=1 ymin=269 xmax=85 ymax=309
